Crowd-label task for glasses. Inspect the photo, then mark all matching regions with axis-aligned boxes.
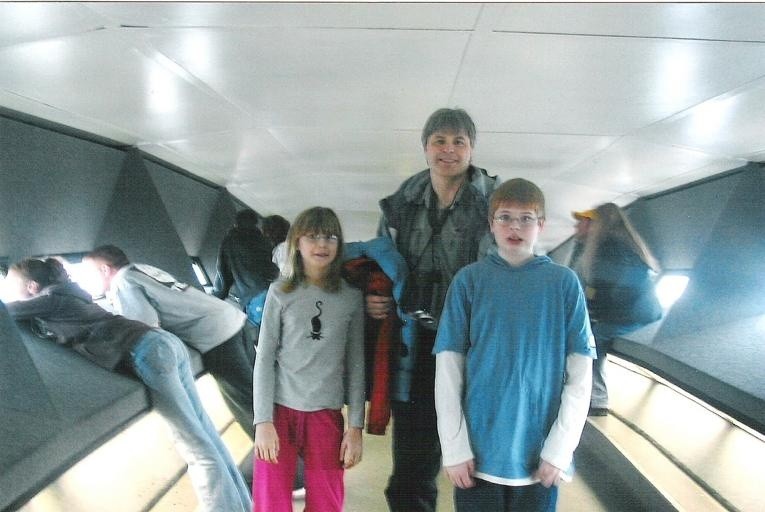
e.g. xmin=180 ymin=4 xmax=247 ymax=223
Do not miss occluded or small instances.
xmin=493 ymin=215 xmax=539 ymax=225
xmin=303 ymin=233 xmax=338 ymax=242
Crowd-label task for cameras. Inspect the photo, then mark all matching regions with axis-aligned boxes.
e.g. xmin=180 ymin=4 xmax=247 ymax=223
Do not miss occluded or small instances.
xmin=400 ymin=269 xmax=442 ymax=325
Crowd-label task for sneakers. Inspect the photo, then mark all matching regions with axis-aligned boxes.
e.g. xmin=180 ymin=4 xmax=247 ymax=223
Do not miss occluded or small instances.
xmin=589 ymin=408 xmax=609 ymax=417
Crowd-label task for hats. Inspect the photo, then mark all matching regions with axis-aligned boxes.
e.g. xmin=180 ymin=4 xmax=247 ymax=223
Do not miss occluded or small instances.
xmin=573 ymin=211 xmax=598 ymax=221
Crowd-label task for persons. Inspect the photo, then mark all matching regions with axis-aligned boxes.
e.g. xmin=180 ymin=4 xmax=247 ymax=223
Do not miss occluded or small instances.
xmin=364 ymin=108 xmax=503 ymax=512
xmin=2 ymin=258 xmax=252 ymax=512
xmin=583 ymin=203 xmax=661 ymax=420
xmin=81 ymin=242 xmax=256 ymax=443
xmin=562 ymin=207 xmax=598 ymax=317
xmin=258 ymin=214 xmax=291 ymax=248
xmin=430 ymin=177 xmax=593 ymax=512
xmin=206 ymin=209 xmax=272 ymax=325
xmin=255 ymin=207 xmax=364 ymax=512
